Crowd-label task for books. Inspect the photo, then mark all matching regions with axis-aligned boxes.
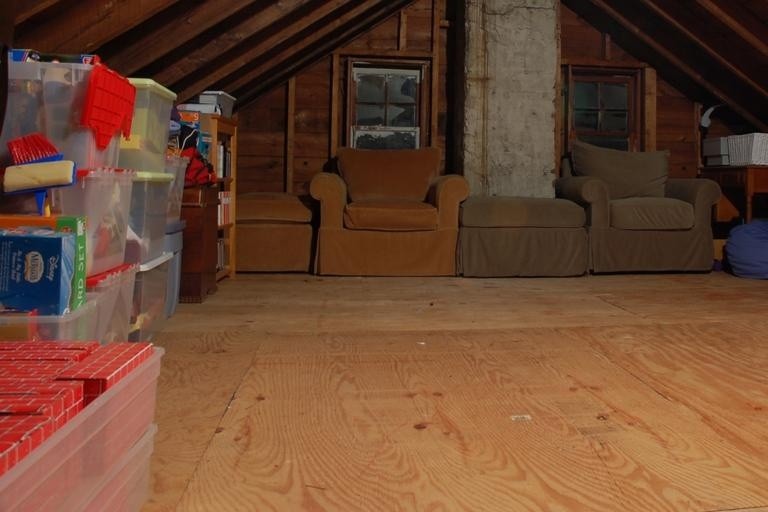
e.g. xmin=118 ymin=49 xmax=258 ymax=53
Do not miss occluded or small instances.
xmin=210 ymin=139 xmax=232 ymax=271
xmin=701 ymin=132 xmax=768 ymax=166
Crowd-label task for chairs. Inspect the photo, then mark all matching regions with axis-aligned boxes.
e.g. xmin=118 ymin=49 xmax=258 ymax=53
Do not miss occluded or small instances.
xmin=311 ymin=159 xmax=469 ymax=276
xmin=556 ymin=158 xmax=721 ymax=273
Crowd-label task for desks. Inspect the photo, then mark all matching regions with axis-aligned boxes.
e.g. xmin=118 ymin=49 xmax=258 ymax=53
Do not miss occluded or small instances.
xmin=697 ymin=166 xmax=768 ymax=222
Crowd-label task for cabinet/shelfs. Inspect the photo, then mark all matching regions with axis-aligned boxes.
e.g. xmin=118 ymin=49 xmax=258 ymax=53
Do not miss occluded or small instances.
xmin=200 ymin=113 xmax=237 ymax=282
xmin=179 ymin=188 xmax=218 ymax=303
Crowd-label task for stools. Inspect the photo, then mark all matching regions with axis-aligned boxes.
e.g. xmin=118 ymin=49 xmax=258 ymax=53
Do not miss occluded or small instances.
xmin=236 ymin=192 xmax=314 ymax=272
xmin=457 ymin=197 xmax=590 ymax=278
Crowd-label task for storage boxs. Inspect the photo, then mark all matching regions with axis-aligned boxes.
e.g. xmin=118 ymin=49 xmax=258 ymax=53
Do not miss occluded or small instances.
xmin=713 ymin=239 xmax=728 ymax=272
xmin=199 ymin=91 xmax=237 ymax=119
xmin=703 ymin=137 xmax=728 ymax=156
xmin=728 ymin=133 xmax=768 ymax=166
xmin=706 ymin=156 xmax=729 ymax=166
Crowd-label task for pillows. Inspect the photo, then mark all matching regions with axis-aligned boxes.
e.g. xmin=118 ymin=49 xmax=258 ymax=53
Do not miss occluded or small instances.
xmin=336 ymin=146 xmax=443 ymax=204
xmin=571 ymin=140 xmax=670 ymax=201
